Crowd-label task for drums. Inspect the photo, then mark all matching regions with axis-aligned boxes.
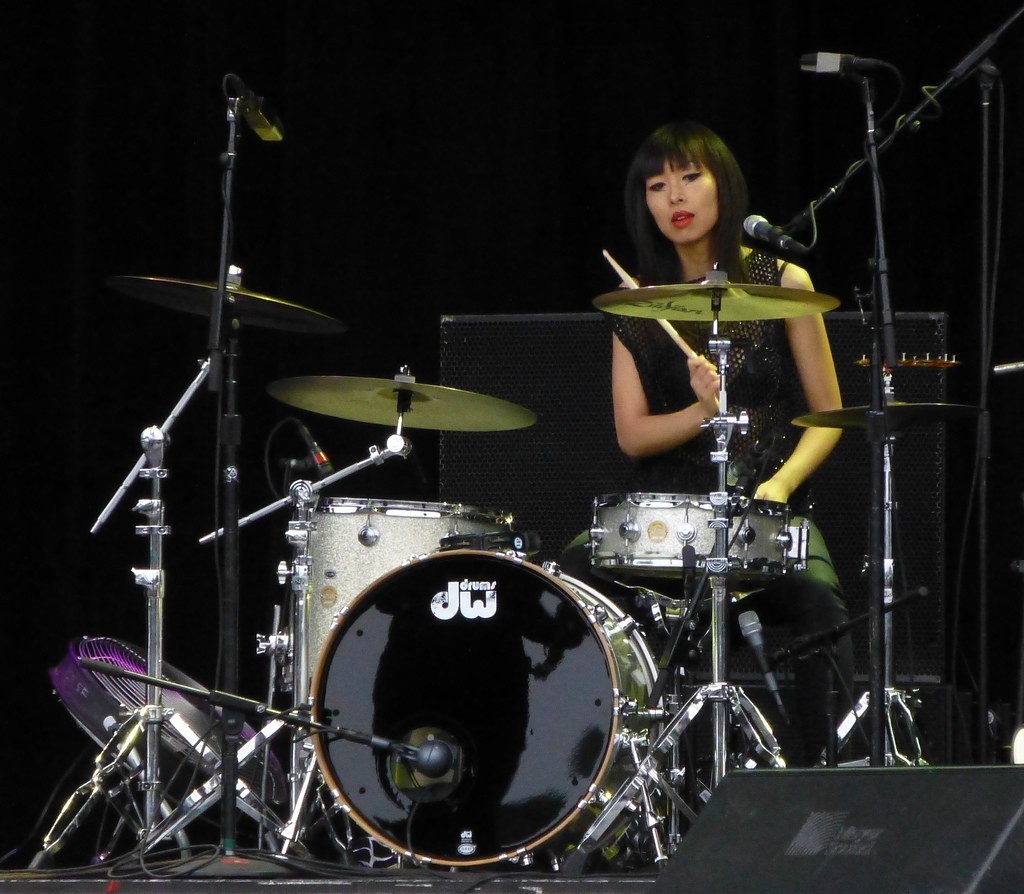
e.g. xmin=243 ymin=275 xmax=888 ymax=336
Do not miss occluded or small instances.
xmin=292 ymin=497 xmax=516 ymax=751
xmin=305 ymin=550 xmax=665 ymax=867
xmin=591 ymin=491 xmax=791 ymax=581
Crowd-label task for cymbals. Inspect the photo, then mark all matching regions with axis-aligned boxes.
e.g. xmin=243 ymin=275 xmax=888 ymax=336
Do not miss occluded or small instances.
xmin=791 ymin=401 xmax=982 ymax=428
xmin=266 ymin=374 xmax=538 ymax=433
xmin=591 ymin=282 xmax=842 ymax=323
xmin=106 ymin=273 xmax=347 ymax=336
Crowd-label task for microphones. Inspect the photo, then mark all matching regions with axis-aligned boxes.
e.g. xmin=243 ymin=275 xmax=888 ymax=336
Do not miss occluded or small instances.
xmin=680 ymin=546 xmax=696 ymax=599
xmin=295 ymin=418 xmax=334 ymax=480
xmin=738 ymin=610 xmax=791 ymax=726
xmin=231 ymin=74 xmax=285 ymax=141
xmin=415 ymin=741 xmax=452 ymax=779
xmin=800 ymin=52 xmax=883 ymax=73
xmin=743 ymin=214 xmax=810 ymax=254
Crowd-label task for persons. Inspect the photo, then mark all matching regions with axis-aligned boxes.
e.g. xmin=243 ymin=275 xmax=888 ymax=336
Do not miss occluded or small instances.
xmin=558 ymin=119 xmax=843 ymax=771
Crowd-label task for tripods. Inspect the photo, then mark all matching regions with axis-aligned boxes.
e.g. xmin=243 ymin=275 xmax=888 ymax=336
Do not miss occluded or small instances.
xmin=817 ymin=430 xmax=921 ymax=769
xmin=564 ymin=325 xmax=788 ymax=876
xmin=29 ymin=327 xmax=416 ymax=875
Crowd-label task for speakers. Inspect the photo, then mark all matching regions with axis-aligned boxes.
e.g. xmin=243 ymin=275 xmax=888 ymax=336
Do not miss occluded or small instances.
xmin=656 ymin=764 xmax=1024 ymax=894
xmin=435 ymin=312 xmax=946 ymax=785
